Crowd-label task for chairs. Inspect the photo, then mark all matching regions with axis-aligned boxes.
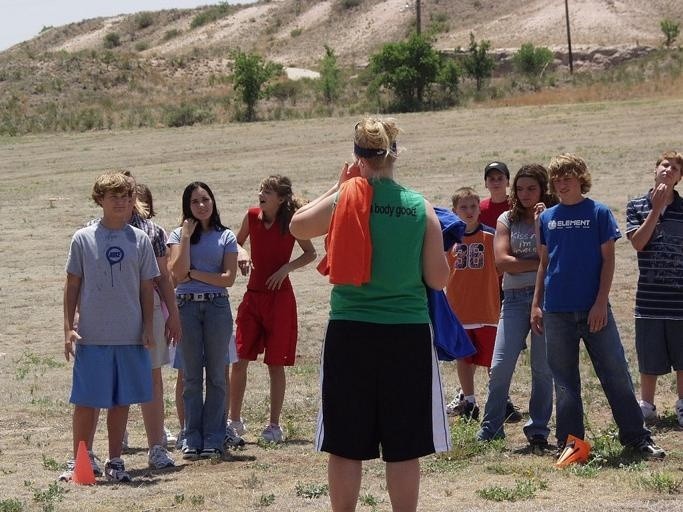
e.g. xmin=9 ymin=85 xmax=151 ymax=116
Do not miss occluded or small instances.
xmin=58 ymin=432 xmax=131 ymax=482
xmin=625 ymin=400 xmax=683 ymax=457
xmin=261 ymin=423 xmax=282 ymax=443
xmin=446 ymin=388 xmax=561 ymax=457
xmin=146 ymin=418 xmax=244 ymax=469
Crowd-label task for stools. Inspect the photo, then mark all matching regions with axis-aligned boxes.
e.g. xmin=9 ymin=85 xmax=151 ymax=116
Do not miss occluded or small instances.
xmin=177 ymin=293 xmax=228 ymax=301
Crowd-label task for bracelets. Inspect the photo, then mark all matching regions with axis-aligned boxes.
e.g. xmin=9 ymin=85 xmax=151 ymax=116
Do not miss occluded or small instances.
xmin=188 ymin=270 xmax=193 ymax=280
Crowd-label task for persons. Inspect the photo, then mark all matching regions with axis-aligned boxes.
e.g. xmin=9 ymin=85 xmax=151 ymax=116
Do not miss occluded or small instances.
xmin=288 ymin=117 xmax=451 ymax=512
xmin=168 ymin=180 xmax=238 ymax=461
xmin=474 ymin=163 xmax=561 ymax=456
xmin=625 ymin=150 xmax=682 ymax=428
xmin=530 ymin=152 xmax=667 ymax=458
xmin=85 ymin=185 xmax=178 ymax=478
xmin=440 ymin=187 xmax=522 ymax=422
xmin=62 ymin=173 xmax=161 ymax=486
xmin=227 ymin=176 xmax=317 ymax=442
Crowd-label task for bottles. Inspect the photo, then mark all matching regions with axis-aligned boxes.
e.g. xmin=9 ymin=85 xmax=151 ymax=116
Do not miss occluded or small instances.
xmin=485 ymin=162 xmax=509 ymax=178
